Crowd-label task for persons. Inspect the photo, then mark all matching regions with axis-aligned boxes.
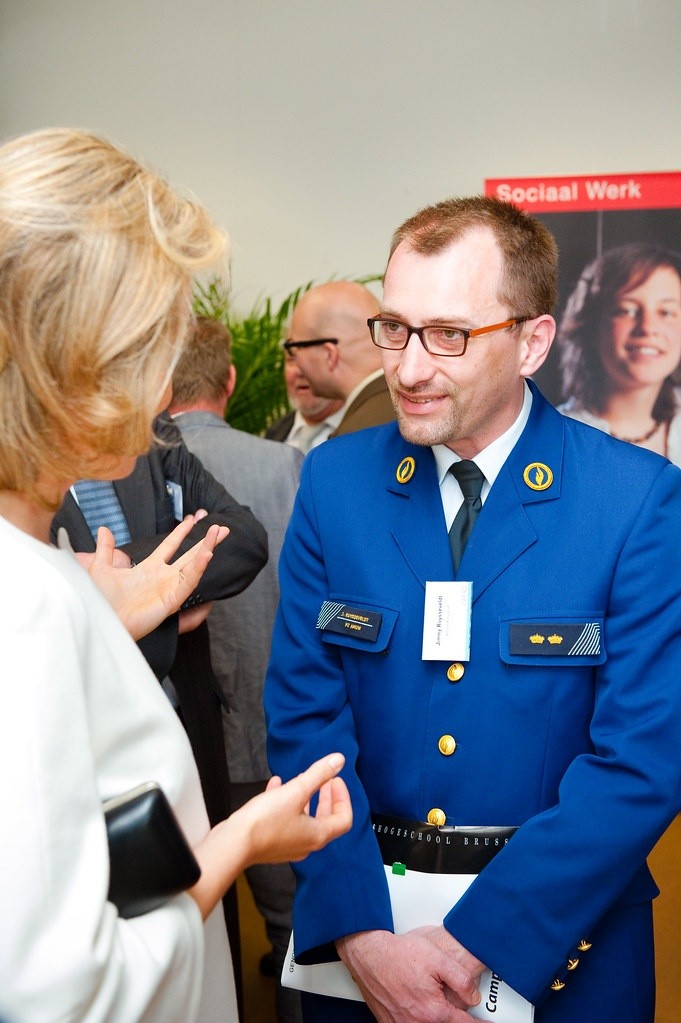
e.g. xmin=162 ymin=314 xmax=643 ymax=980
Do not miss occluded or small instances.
xmin=42 ymin=406 xmax=269 ymax=991
xmin=266 ymin=330 xmax=342 ymax=453
xmin=546 ymin=241 xmax=680 ymax=466
xmin=2 ymin=126 xmax=354 ymax=1022
xmin=156 ymin=320 xmax=305 ymax=979
xmin=286 ymin=280 xmax=401 ymax=440
xmin=261 ymin=193 xmax=680 ymax=1023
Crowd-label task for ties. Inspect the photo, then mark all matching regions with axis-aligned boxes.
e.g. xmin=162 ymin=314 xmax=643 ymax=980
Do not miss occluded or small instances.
xmin=440 ymin=458 xmax=485 ymax=579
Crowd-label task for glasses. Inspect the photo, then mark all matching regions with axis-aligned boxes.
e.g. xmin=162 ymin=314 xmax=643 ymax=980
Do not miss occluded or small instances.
xmin=284 ymin=338 xmax=338 ymax=354
xmin=365 ymin=316 xmax=531 ymax=356
